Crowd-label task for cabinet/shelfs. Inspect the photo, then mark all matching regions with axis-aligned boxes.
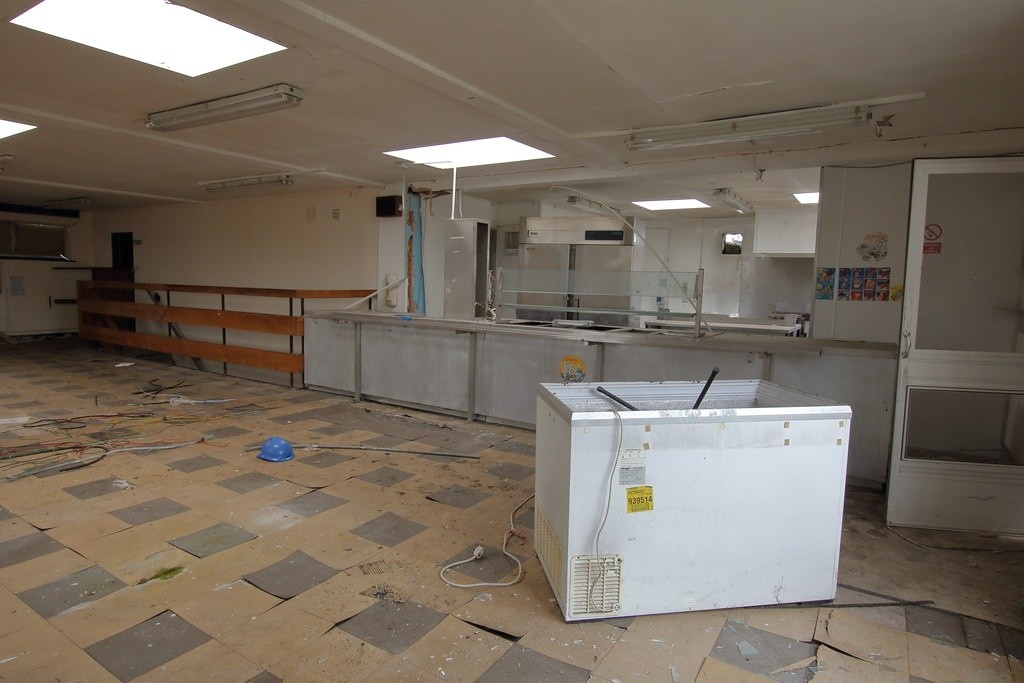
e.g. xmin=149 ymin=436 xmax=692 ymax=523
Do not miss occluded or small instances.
xmin=0 ymin=260 xmax=97 ymax=336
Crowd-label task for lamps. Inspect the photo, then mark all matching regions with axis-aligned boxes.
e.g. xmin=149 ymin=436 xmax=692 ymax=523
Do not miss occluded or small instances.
xmin=197 ymin=172 xmax=296 ymax=193
xmin=43 ymin=198 xmax=96 ymax=211
xmin=147 ymin=82 xmax=305 ymax=133
xmin=622 ymin=102 xmax=871 ymax=152
xmin=566 ymin=196 xmax=620 ymax=218
xmin=0 ymin=154 xmax=15 ymax=176
xmin=713 ymin=188 xmax=753 ymax=215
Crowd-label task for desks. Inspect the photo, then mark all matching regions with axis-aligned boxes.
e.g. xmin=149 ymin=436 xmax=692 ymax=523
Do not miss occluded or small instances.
xmin=644 ymin=320 xmax=802 ymax=337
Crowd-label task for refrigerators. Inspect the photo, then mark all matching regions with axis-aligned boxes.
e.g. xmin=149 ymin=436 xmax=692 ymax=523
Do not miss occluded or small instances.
xmin=535 ymin=379 xmax=852 ymax=622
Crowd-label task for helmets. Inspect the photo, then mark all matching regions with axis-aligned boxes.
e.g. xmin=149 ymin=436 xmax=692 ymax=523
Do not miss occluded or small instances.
xmin=256 ymin=437 xmax=296 ymax=463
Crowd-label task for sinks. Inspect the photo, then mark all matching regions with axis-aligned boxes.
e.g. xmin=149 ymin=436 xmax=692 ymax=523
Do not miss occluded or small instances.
xmin=575 ymin=326 xmax=623 ymax=332
xmin=541 ymin=324 xmax=574 ymax=328
xmin=619 ymin=328 xmax=658 ymax=334
xmin=498 ymin=321 xmax=554 ymax=326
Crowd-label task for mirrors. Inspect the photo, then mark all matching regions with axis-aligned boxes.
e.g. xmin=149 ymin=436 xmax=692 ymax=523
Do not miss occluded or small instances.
xmin=407 ymin=164 xmax=824 ymax=338
